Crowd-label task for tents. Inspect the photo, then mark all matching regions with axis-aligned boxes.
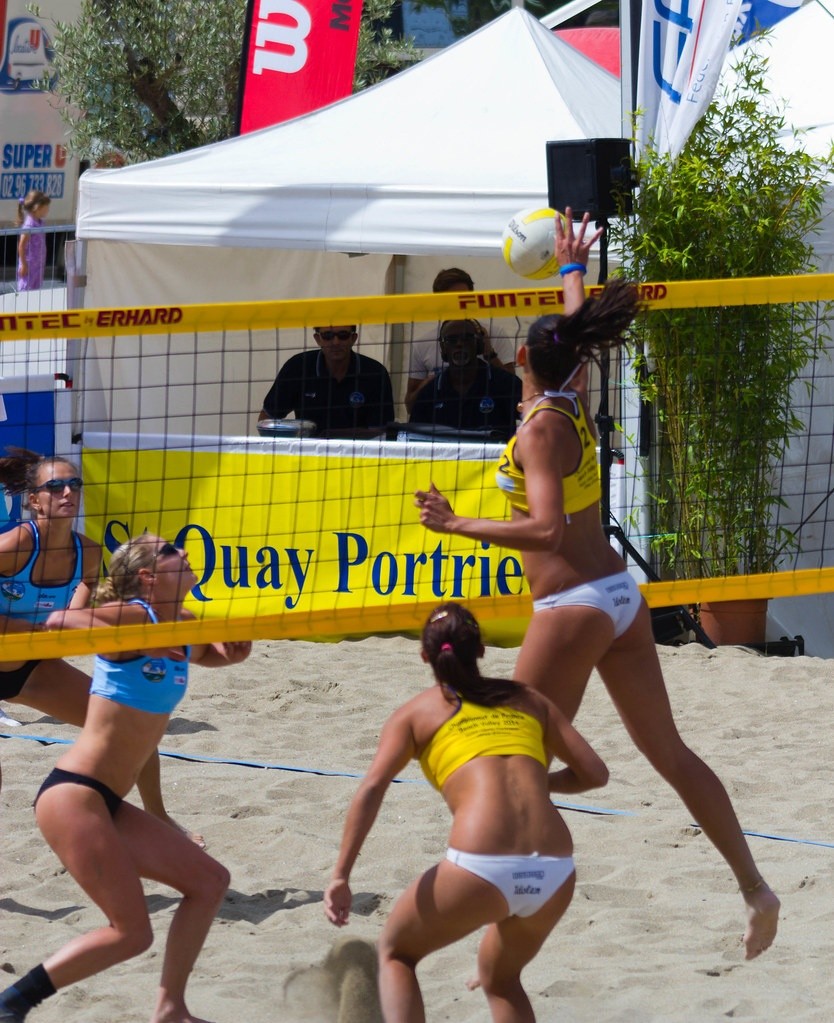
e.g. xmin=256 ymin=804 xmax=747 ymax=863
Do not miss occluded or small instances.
xmin=63 ymin=4 xmax=834 ymax=658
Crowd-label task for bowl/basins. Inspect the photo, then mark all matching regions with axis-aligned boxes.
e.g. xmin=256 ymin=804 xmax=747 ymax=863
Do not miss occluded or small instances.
xmin=257 ymin=418 xmax=315 ymax=437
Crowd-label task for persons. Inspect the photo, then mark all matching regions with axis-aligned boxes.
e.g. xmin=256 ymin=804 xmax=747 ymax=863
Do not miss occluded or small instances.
xmin=1 ymin=535 xmax=254 ymax=1022
xmin=318 ymin=599 xmax=611 ymax=1023
xmin=14 ymin=190 xmax=52 ymax=293
xmin=0 ymin=444 xmax=211 ymax=851
xmin=412 ymin=207 xmax=781 ymax=964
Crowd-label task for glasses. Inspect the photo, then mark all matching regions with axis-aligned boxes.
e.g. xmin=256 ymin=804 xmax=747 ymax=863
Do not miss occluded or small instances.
xmin=316 ymin=329 xmax=355 ymax=341
xmin=144 ymin=541 xmax=177 ymax=569
xmin=34 ymin=477 xmax=84 ymax=493
xmin=442 ymin=333 xmax=477 ymax=342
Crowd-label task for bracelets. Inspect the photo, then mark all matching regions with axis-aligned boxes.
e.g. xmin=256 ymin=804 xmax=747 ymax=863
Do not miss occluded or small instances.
xmin=559 ymin=263 xmax=587 ymax=277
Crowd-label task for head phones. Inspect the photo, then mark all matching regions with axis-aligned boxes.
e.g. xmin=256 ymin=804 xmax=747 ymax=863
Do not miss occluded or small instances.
xmin=437 ymin=319 xmax=486 ymax=363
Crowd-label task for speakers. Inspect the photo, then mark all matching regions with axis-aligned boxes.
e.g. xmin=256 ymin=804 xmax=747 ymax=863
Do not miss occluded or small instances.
xmin=546 ymin=136 xmax=634 ymax=222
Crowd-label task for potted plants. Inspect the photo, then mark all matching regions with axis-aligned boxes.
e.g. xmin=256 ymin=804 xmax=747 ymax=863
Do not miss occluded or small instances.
xmin=601 ymin=36 xmax=834 ymax=648
